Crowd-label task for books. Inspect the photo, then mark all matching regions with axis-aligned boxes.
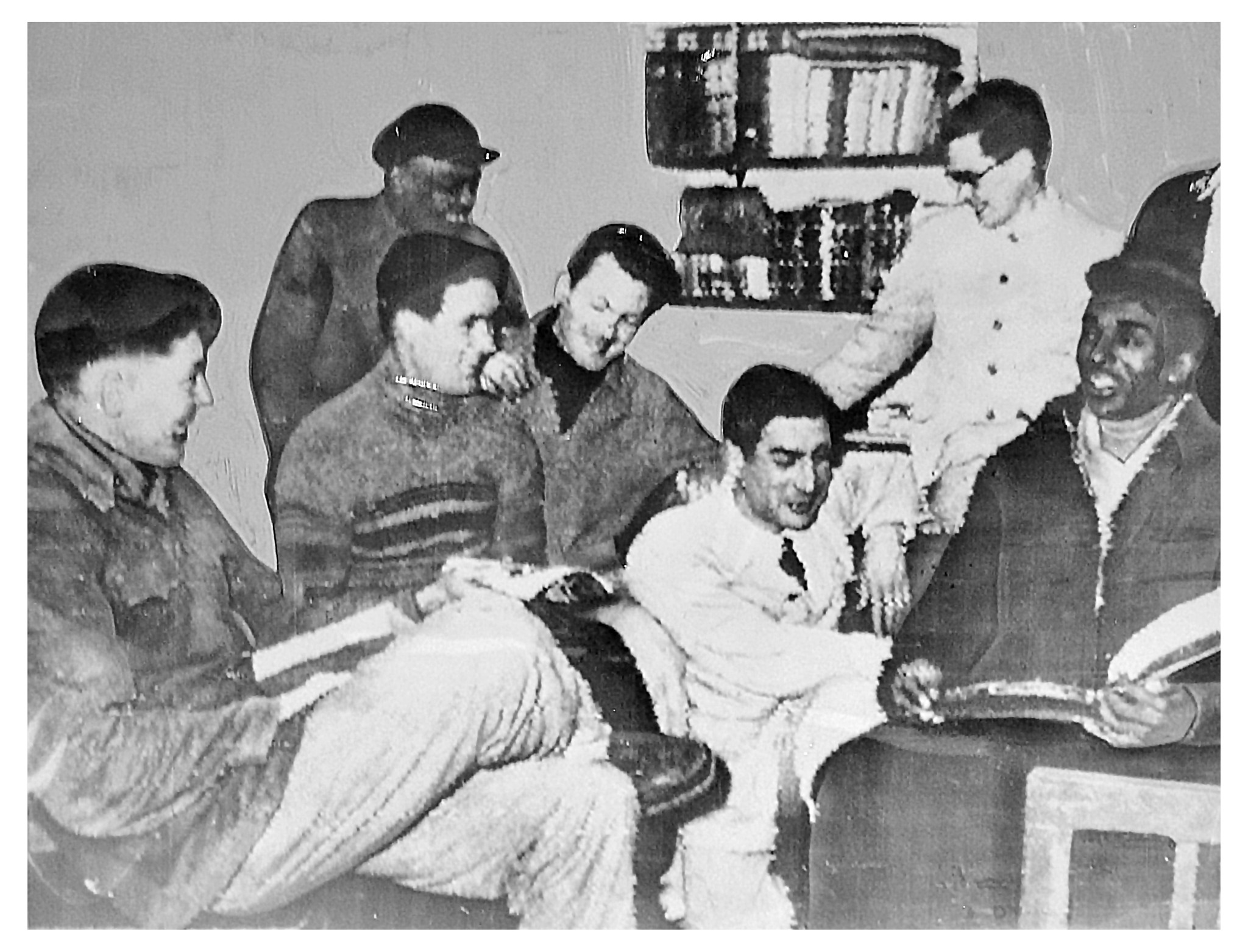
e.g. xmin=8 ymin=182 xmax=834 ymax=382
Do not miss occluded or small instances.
xmin=938 ymin=581 xmax=1219 ymax=722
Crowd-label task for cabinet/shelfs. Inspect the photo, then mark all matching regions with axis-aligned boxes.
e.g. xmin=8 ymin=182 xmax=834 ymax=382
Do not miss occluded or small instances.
xmin=666 ymin=152 xmax=945 ymax=312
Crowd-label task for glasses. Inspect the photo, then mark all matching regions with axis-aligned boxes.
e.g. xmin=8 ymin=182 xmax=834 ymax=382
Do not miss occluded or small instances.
xmin=943 ymin=150 xmax=1020 ymax=190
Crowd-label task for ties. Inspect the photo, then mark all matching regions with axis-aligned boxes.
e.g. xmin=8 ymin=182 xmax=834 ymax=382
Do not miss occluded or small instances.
xmin=779 ymin=535 xmax=807 ymax=601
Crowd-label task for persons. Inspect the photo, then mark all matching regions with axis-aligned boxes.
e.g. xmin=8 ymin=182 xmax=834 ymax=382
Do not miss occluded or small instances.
xmin=28 ymin=76 xmax=1222 ymax=929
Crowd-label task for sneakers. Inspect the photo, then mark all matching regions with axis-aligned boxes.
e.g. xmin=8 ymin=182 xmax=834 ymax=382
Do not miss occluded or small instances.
xmin=606 ymin=724 xmax=718 ymax=818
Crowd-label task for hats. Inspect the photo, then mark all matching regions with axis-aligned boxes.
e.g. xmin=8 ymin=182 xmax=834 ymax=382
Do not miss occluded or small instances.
xmin=372 ymin=104 xmax=501 ymax=169
